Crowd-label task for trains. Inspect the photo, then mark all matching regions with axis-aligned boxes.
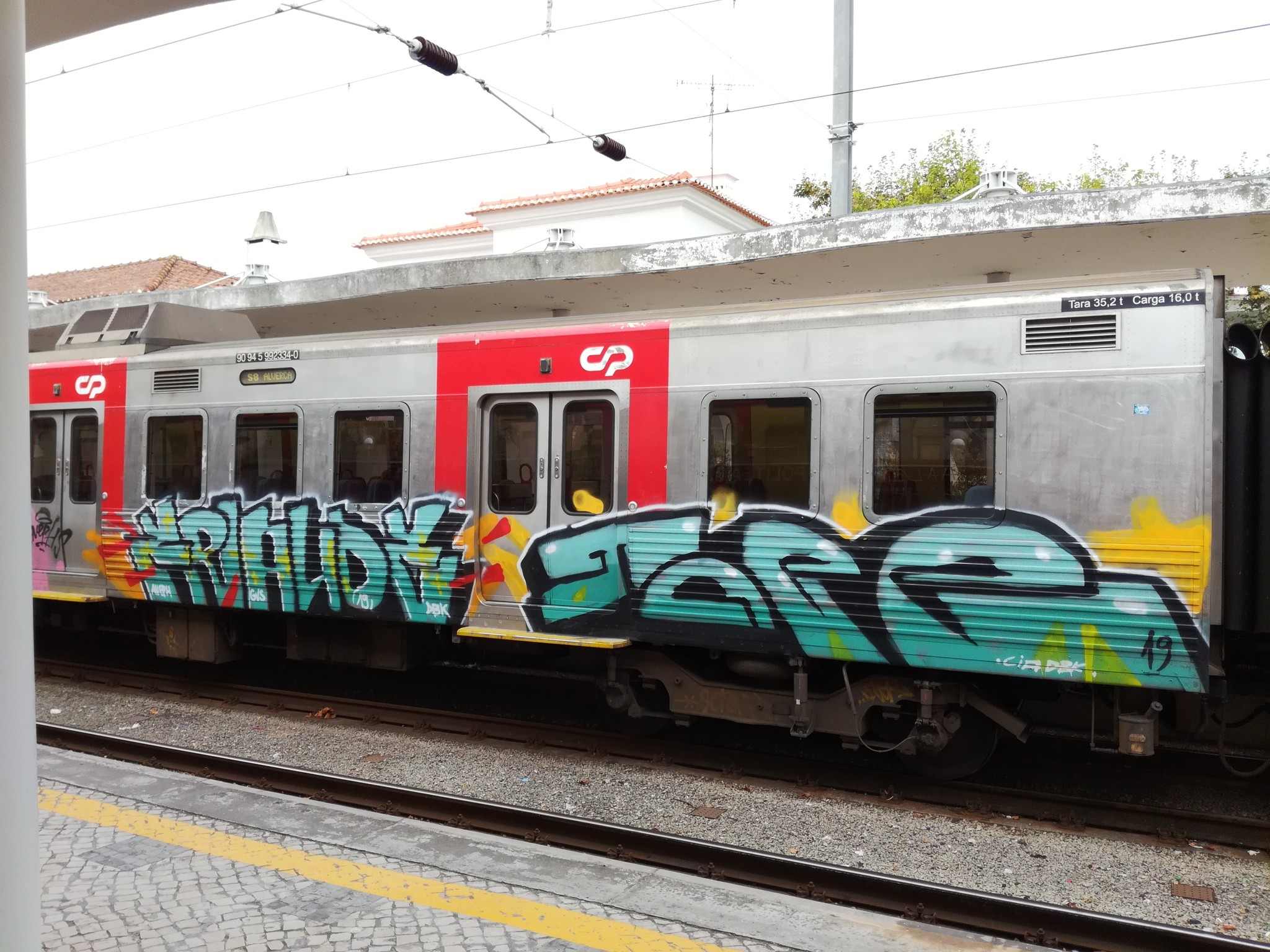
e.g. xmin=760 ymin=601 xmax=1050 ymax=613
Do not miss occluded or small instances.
xmin=29 ymin=267 xmax=1269 ymax=791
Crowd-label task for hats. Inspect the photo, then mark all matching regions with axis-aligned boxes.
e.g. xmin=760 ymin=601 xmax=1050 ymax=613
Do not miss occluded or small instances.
xmin=364 ymin=438 xmax=373 ymax=444
xmin=950 ymin=439 xmax=966 ymax=445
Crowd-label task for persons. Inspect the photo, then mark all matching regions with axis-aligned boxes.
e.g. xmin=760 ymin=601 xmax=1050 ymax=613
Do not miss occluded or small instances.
xmin=732 ymin=449 xmax=766 ymax=501
